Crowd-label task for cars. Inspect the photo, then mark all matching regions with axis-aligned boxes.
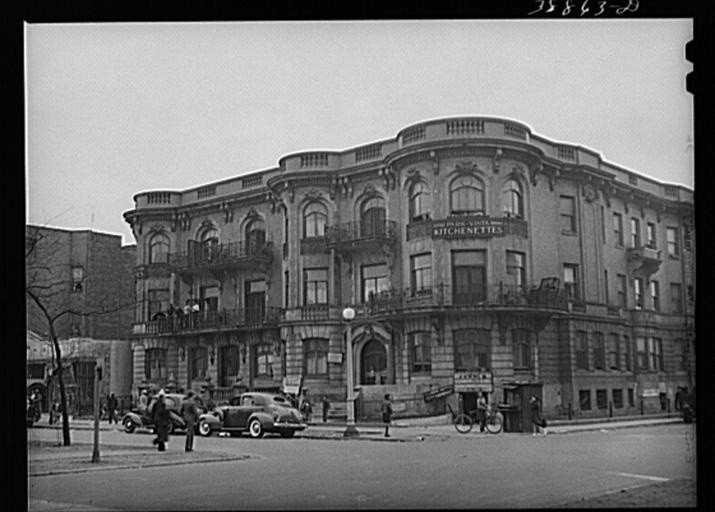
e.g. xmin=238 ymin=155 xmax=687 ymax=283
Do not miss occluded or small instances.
xmin=122 ymin=393 xmax=204 ymax=434
xmin=196 ymin=392 xmax=308 ymax=438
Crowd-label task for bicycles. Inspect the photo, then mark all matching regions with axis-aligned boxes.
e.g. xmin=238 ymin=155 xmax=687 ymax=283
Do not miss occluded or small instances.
xmin=454 ymin=403 xmax=503 ymax=434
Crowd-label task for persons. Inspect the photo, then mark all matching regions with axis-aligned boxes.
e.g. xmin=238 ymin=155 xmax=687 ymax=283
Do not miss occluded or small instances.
xmin=291 ymin=392 xmax=299 ymax=409
xmin=107 ymin=392 xmax=118 ymax=425
xmin=475 ymin=390 xmax=487 ymax=431
xmin=380 ymin=394 xmax=394 ymax=436
xmin=32 ymin=389 xmax=41 ymax=422
xmin=527 ymin=394 xmax=547 ymax=437
xmin=151 ymin=394 xmax=166 ymax=434
xmin=29 ymin=389 xmax=36 ymax=400
xmin=49 ymin=399 xmax=61 ymax=425
xmin=321 ymin=394 xmax=330 ymax=423
xmin=152 ymin=402 xmax=170 ymax=450
xmin=300 ymin=395 xmax=313 ymax=421
xmin=675 ymin=385 xmax=685 ymax=413
xmin=139 ymin=388 xmax=148 ymax=409
xmin=181 ymin=390 xmax=199 ymax=451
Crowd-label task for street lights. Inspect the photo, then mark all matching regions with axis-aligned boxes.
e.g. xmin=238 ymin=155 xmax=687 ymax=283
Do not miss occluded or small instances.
xmin=342 ymin=307 xmax=360 ymax=437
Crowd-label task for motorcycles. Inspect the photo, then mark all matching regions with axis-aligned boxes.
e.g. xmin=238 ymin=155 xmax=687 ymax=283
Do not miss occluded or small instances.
xmin=682 ymin=400 xmax=695 ymax=424
